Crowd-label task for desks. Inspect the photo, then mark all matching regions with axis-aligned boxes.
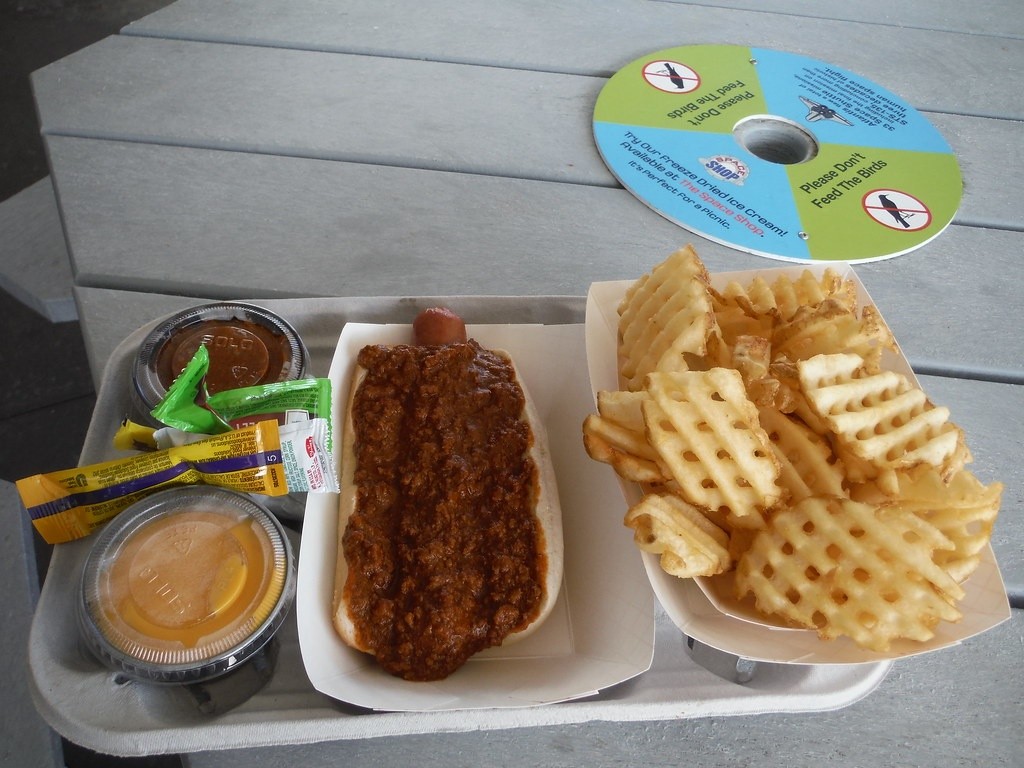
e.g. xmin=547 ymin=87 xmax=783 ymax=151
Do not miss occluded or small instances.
xmin=0 ymin=0 xmax=1024 ymax=768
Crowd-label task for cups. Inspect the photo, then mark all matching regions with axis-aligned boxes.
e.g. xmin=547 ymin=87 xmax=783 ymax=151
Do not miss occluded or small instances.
xmin=128 ymin=302 xmax=311 ymax=433
xmin=74 ymin=482 xmax=297 ymax=723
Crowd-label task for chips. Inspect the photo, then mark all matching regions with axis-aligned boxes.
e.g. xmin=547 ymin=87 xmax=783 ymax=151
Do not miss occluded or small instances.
xmin=581 ymin=244 xmax=1004 ymax=655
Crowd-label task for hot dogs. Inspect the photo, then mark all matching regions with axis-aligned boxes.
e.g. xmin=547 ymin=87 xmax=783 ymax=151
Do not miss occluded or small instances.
xmin=335 ymin=306 xmax=566 ymax=681
